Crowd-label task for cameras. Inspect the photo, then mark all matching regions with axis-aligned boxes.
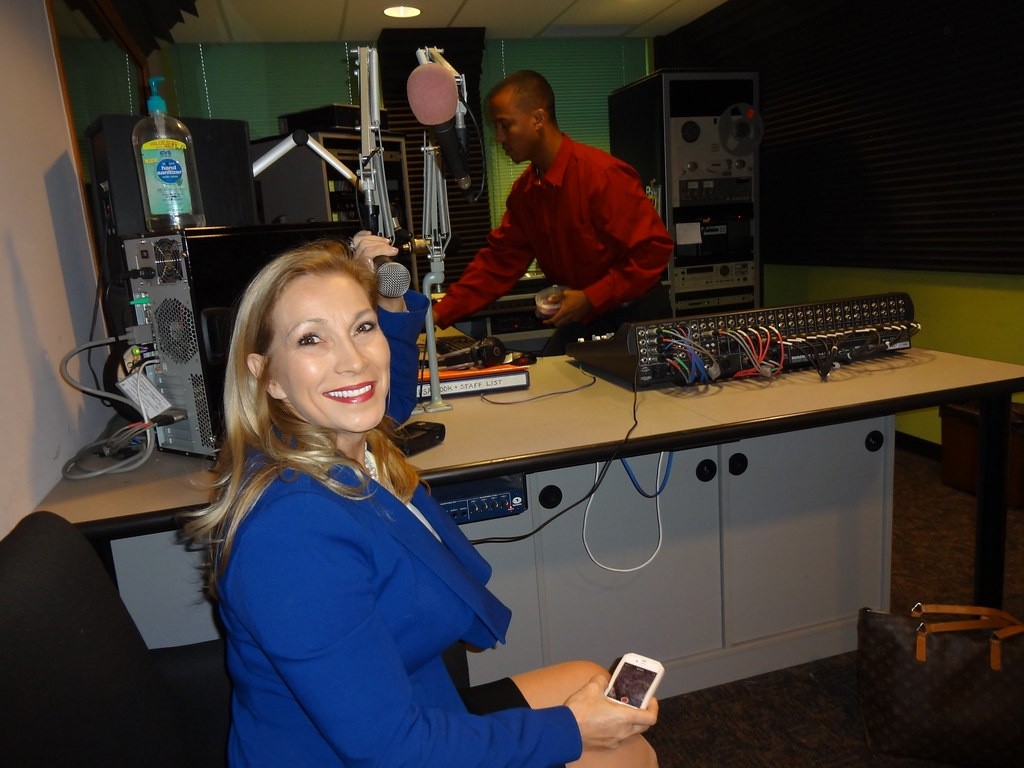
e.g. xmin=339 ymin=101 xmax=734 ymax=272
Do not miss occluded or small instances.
xmin=392 ymin=421 xmax=445 ymax=454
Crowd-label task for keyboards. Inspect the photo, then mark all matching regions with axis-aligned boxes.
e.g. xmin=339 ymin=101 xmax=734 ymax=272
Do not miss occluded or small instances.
xmin=435 ymin=336 xmax=477 ymax=355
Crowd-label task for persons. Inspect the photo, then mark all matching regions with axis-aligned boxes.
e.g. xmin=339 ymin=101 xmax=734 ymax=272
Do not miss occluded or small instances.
xmin=421 ymin=69 xmax=674 ymax=357
xmin=177 ymin=230 xmax=658 ymax=768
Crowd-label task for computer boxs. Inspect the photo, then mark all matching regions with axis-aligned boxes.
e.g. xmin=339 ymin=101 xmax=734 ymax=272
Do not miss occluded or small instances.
xmin=121 ymin=222 xmax=365 ymax=461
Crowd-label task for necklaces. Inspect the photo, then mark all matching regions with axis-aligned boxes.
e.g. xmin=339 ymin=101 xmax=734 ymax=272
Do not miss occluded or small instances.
xmin=365 ymin=456 xmax=377 ymax=482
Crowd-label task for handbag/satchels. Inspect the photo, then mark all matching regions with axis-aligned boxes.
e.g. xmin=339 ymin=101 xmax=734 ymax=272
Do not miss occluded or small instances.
xmin=859 ymin=604 xmax=1024 ymax=765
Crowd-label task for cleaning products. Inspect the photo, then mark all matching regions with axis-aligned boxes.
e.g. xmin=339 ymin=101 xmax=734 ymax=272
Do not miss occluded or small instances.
xmin=131 ymin=76 xmax=206 ymax=233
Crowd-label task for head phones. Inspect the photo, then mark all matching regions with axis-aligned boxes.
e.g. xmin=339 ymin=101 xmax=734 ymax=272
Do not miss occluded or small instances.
xmin=418 ymin=337 xmax=506 ymax=369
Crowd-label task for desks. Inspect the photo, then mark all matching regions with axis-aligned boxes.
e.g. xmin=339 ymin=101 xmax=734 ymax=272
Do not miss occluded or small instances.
xmin=33 ymin=346 xmax=1024 ymax=701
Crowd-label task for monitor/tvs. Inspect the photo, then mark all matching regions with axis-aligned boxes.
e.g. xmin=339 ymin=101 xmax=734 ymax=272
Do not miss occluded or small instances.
xmin=250 ymin=132 xmax=333 ymax=223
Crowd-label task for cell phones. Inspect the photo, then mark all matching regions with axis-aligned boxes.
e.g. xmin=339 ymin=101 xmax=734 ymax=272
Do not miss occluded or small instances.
xmin=604 ymin=653 xmax=664 ymax=711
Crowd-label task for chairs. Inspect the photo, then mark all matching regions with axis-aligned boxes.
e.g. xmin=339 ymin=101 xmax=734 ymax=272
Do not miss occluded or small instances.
xmin=0 ymin=510 xmax=237 ymax=767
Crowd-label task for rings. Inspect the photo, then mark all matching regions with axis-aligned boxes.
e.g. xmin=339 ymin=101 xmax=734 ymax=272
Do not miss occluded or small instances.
xmin=348 ymin=239 xmax=356 ymax=250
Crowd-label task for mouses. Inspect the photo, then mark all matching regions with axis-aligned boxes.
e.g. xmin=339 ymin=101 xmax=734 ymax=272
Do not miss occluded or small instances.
xmin=511 ymin=352 xmax=537 ymax=366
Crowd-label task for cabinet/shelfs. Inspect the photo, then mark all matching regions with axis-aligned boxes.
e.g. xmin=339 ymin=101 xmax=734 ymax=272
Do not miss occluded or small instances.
xmin=252 ymin=133 xmax=419 ymax=293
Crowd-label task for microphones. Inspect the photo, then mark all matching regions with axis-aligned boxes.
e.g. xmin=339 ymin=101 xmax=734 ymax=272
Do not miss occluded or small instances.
xmin=408 ymin=64 xmax=476 ymax=204
xmin=371 ymin=255 xmax=411 ymax=298
